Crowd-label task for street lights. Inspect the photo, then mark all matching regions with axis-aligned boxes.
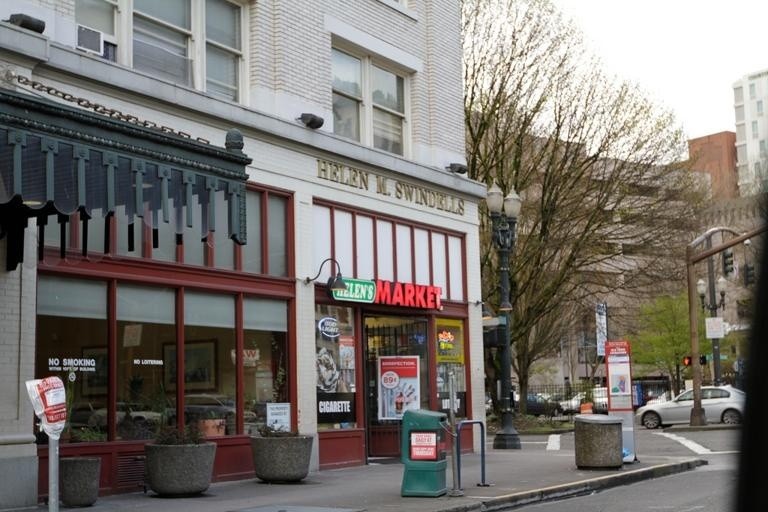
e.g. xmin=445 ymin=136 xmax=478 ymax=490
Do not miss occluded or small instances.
xmin=696 ymin=276 xmax=730 ymax=386
xmin=487 ymin=178 xmax=524 ymax=450
xmin=687 ymin=226 xmax=752 ymax=425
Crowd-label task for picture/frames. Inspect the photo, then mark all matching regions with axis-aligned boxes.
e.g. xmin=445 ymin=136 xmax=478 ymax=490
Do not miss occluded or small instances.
xmin=81 ymin=342 xmax=133 ymax=401
xmin=157 ymin=338 xmax=219 ymax=394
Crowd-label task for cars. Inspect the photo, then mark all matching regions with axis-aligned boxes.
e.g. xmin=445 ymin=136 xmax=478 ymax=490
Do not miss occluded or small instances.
xmin=636 ymin=385 xmax=746 ymax=429
xmin=88 ymin=401 xmax=163 ymax=430
xmin=486 ymin=384 xmax=608 ymax=417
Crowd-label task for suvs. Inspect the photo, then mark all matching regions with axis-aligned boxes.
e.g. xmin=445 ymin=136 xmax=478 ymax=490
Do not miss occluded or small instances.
xmin=166 ymin=393 xmax=257 ymax=429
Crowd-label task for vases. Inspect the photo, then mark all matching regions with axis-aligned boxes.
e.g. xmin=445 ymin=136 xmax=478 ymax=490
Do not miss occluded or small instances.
xmin=57 ymin=456 xmax=103 ymax=508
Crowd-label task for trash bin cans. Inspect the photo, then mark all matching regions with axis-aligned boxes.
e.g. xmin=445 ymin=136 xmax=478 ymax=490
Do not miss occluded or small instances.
xmin=580 ymin=403 xmax=594 ymax=413
xmin=401 ymin=409 xmax=448 ymax=498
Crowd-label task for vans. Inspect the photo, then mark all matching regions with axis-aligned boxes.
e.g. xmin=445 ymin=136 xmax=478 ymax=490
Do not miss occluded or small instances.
xmin=68 ymin=400 xmax=103 ymax=425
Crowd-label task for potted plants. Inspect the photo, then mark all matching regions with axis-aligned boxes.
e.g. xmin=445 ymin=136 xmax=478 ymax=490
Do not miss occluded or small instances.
xmin=142 ymin=425 xmax=218 ymax=496
xmin=247 ymin=419 xmax=315 ymax=483
xmin=196 ymin=408 xmax=225 ymax=437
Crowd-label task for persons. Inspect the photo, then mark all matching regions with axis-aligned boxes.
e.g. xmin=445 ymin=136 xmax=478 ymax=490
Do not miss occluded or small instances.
xmin=564 ymin=377 xmax=571 ymax=400
xmin=510 ymin=385 xmax=517 ymax=408
xmin=580 ymin=391 xmax=598 ymax=414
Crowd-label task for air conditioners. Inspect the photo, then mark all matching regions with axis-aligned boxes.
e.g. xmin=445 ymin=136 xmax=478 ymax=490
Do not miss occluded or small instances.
xmin=74 ymin=24 xmax=106 ymax=56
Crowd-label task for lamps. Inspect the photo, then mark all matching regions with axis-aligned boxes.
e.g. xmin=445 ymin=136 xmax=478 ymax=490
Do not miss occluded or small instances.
xmin=475 ymin=284 xmax=514 ymax=312
xmin=445 ymin=161 xmax=470 ymax=176
xmin=294 ymin=113 xmax=324 ymax=130
xmin=4 ymin=14 xmax=46 ymax=35
xmin=300 ymin=256 xmax=349 ymax=293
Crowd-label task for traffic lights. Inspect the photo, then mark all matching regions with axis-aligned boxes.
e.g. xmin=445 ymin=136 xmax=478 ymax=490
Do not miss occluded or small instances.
xmin=684 ymin=357 xmax=691 ymax=364
xmin=723 ymin=251 xmax=733 ymax=274
xmin=745 ymin=264 xmax=755 ymax=286
